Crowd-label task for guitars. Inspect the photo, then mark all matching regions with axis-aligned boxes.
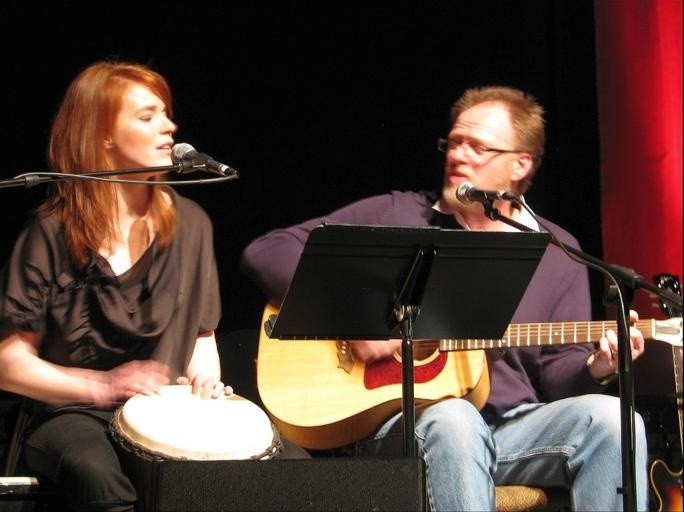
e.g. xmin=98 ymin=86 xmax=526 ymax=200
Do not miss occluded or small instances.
xmin=649 ymin=272 xmax=683 ymax=511
xmin=256 ymin=296 xmax=684 ymax=449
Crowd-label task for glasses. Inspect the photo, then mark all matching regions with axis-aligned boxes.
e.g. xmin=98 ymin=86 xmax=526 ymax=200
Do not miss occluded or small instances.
xmin=438 ymin=138 xmax=523 ymax=154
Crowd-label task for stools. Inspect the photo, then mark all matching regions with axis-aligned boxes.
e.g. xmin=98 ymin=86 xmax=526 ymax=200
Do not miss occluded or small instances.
xmin=497 ymin=487 xmax=556 ymax=511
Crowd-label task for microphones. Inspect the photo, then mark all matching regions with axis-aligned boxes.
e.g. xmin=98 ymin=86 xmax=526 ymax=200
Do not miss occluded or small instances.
xmin=455 ymin=180 xmax=515 ymax=206
xmin=170 ymin=143 xmax=240 ymax=179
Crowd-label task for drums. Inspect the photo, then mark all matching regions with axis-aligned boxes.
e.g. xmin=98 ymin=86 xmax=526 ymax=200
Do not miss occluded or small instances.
xmin=109 ymin=385 xmax=282 ymax=510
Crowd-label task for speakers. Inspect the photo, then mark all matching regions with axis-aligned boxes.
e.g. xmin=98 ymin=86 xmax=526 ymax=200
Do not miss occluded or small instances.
xmin=155 ymin=456 xmax=429 ymax=512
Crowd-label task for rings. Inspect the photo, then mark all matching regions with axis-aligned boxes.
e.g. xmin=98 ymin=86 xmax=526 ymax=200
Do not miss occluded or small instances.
xmin=611 ymin=350 xmax=618 ymax=354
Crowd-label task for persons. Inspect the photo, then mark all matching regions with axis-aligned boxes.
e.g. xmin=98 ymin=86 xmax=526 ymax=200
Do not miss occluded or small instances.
xmin=0 ymin=58 xmax=314 ymax=512
xmin=243 ymin=82 xmax=653 ymax=512
